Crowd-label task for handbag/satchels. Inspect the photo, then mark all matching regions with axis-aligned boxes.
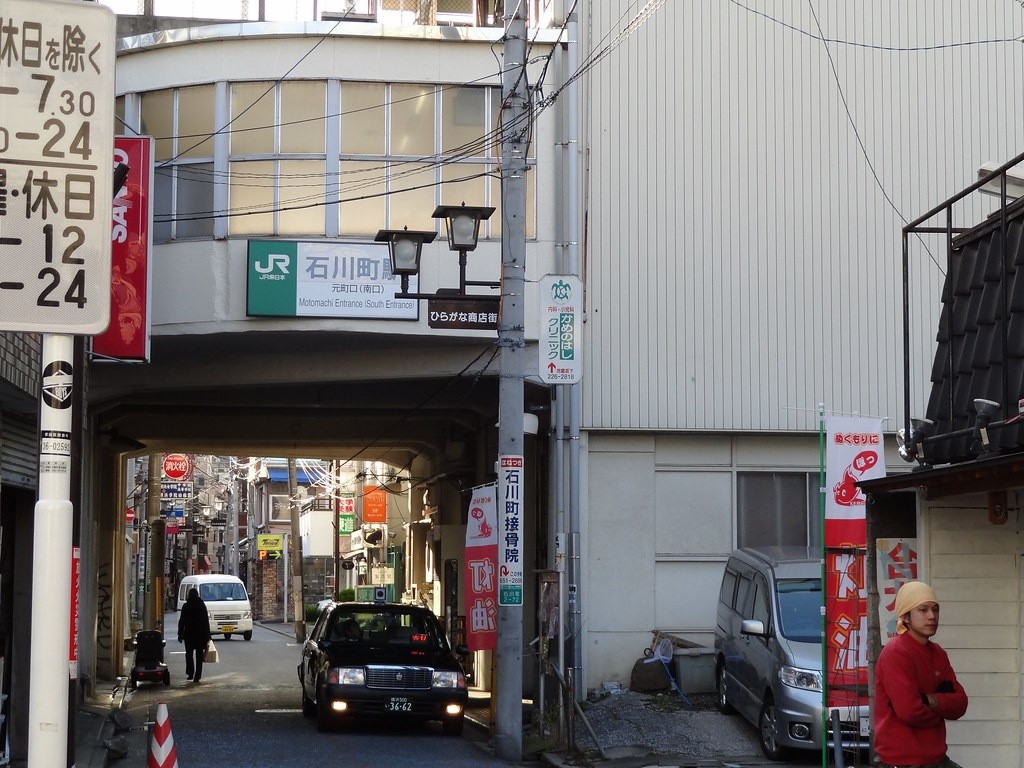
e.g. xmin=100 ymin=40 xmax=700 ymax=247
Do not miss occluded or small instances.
xmin=203 ymin=639 xmax=220 ymax=664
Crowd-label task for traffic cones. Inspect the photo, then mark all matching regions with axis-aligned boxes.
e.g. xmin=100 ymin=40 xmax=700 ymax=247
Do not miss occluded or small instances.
xmin=147 ymin=703 xmax=179 ymax=768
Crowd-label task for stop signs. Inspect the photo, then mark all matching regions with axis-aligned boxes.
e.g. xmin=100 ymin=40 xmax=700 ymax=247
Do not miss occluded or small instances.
xmin=127 ymin=509 xmax=134 ymax=521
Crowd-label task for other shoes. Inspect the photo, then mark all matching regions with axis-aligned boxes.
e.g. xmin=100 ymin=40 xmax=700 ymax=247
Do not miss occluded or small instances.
xmin=193 ymin=678 xmax=199 ymax=682
xmin=187 ymin=674 xmax=193 ymax=680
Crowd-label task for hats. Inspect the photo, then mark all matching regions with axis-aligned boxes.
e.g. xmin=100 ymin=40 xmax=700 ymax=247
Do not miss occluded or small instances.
xmin=187 ymin=588 xmax=199 ymax=597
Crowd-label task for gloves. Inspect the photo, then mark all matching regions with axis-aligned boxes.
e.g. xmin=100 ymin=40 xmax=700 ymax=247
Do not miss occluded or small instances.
xmin=935 ymin=681 xmax=954 ymax=692
xmin=888 ymin=693 xmax=938 ymax=709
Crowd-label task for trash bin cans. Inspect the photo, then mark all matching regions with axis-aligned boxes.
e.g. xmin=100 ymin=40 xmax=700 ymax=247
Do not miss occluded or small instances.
xmin=672 ymin=648 xmax=718 ymax=694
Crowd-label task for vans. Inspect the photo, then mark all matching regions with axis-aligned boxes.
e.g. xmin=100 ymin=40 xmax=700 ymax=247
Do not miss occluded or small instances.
xmin=714 ymin=545 xmax=871 ymax=761
xmin=177 ymin=574 xmax=253 ymax=641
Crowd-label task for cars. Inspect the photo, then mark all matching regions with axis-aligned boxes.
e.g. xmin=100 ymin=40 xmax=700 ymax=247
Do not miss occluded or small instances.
xmin=300 ymin=600 xmax=468 ymax=737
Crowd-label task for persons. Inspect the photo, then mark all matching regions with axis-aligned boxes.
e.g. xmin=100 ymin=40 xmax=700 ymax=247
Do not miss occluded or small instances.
xmin=873 ymin=580 xmax=968 ymax=768
xmin=177 ymin=588 xmax=212 ymax=682
xmin=202 ymin=586 xmax=212 ymax=599
